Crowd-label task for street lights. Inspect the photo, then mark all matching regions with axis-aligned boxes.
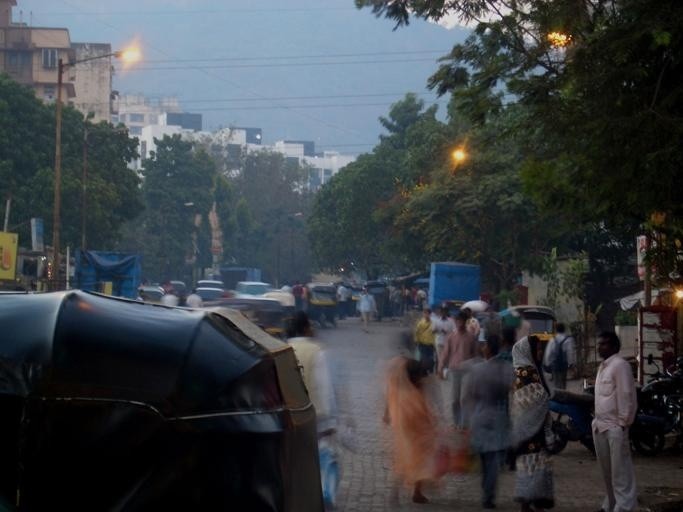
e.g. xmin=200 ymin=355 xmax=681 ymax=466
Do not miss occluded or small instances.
xmin=51 ymin=43 xmax=142 ymax=299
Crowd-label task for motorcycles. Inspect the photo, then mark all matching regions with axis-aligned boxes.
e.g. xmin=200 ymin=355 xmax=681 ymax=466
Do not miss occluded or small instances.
xmin=546 ymin=354 xmax=681 ymax=457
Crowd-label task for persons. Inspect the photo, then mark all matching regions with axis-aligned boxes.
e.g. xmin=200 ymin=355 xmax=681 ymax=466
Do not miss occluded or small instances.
xmin=276 ymin=282 xmax=640 ymax=512
xmin=134 ymin=279 xmax=204 ymax=309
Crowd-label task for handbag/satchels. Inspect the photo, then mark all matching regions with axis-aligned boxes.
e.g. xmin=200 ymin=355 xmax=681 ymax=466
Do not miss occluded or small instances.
xmin=318 ymin=430 xmax=344 ymax=506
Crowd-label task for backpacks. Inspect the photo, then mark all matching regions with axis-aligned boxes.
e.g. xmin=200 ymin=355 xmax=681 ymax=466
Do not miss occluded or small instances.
xmin=549 ymin=335 xmax=571 ymax=380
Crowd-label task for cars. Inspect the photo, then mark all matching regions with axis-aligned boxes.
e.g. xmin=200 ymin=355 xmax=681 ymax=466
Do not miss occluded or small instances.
xmin=136 ymin=277 xmax=340 ymax=341
